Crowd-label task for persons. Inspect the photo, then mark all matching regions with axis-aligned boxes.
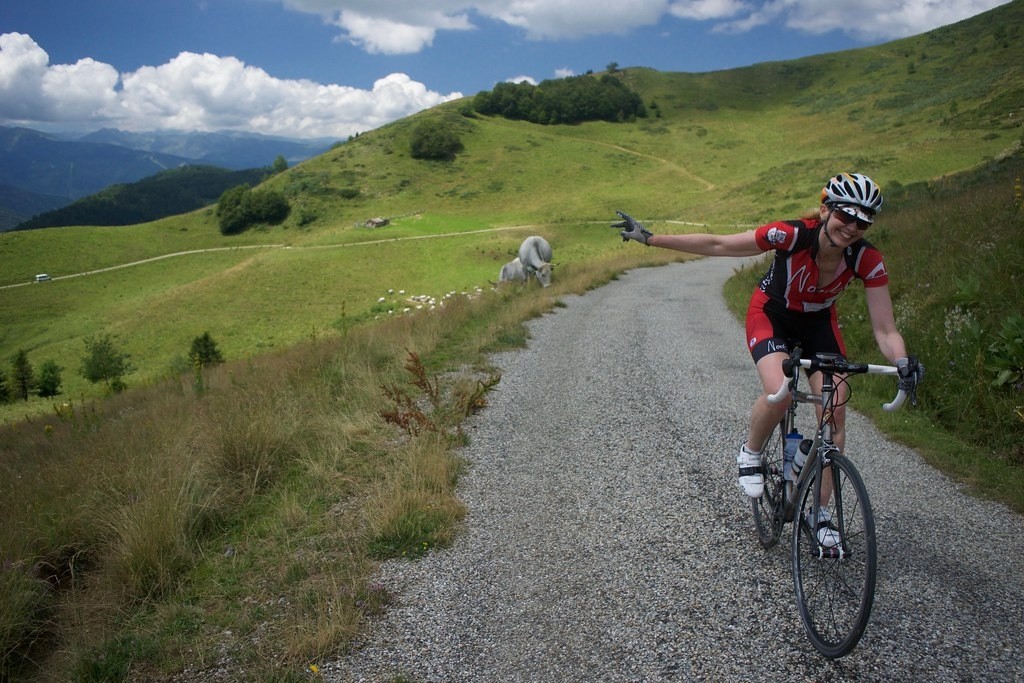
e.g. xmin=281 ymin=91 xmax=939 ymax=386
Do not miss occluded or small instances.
xmin=612 ymin=173 xmax=924 ymax=547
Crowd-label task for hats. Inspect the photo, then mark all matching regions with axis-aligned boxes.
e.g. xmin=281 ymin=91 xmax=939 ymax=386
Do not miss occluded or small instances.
xmin=832 ymin=202 xmax=875 ymax=224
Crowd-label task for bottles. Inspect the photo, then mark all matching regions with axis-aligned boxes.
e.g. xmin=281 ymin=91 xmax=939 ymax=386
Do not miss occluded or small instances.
xmin=784 ymin=428 xmax=803 ymax=480
xmin=789 ymin=439 xmax=813 ymax=482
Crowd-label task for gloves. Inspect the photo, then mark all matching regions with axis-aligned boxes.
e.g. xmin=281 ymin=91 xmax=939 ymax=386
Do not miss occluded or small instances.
xmin=609 ymin=210 xmax=653 ymax=247
xmin=896 ymin=357 xmax=925 ymax=407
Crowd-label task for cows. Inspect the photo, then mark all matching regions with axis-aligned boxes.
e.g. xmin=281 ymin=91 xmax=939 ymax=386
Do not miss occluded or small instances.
xmin=487 ymin=236 xmax=562 ymax=290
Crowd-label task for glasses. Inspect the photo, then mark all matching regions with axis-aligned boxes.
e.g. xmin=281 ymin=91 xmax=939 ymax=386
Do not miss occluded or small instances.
xmin=833 ymin=212 xmax=870 ymax=230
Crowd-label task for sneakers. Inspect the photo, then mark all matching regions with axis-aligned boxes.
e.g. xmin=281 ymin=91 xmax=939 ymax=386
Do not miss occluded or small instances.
xmin=737 ymin=440 xmax=764 ymax=499
xmin=806 ymin=506 xmax=840 ymax=548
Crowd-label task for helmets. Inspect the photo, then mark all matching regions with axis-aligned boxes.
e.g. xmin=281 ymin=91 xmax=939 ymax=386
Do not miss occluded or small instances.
xmin=821 ymin=172 xmax=884 ymax=224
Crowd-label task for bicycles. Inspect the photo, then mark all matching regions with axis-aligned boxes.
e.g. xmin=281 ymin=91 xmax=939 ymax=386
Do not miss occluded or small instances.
xmin=751 ymin=345 xmax=919 ymax=662
